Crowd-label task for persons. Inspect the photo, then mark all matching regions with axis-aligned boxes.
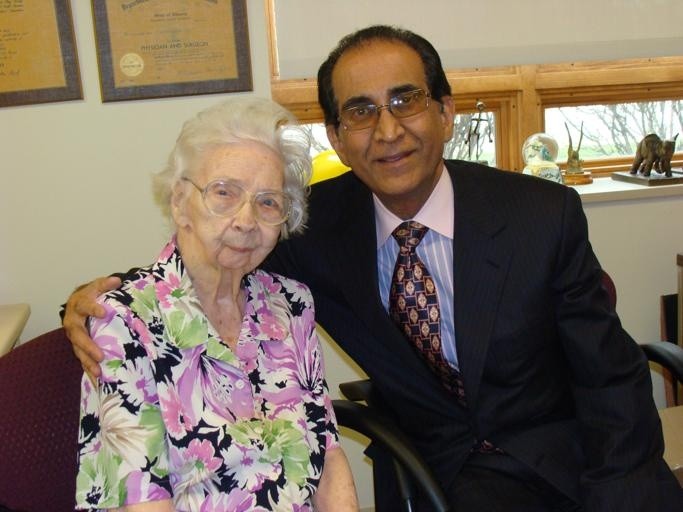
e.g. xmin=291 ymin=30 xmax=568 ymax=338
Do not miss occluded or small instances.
xmin=58 ymin=25 xmax=681 ymax=510
xmin=74 ymin=92 xmax=362 ymax=510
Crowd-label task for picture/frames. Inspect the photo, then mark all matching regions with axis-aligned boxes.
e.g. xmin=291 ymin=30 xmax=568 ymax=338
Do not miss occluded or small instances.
xmin=91 ymin=0 xmax=254 ymax=102
xmin=0 ymin=0 xmax=83 ymax=107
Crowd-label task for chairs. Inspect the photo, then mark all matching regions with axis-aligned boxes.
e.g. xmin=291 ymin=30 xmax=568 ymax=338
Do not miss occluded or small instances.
xmin=0 ymin=269 xmax=683 ymax=512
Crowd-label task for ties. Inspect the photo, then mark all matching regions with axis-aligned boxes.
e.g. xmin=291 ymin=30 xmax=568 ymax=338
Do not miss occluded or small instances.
xmin=388 ymin=222 xmax=501 ymax=453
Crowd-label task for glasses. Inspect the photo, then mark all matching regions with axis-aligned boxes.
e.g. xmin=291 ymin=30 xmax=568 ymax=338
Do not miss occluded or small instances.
xmin=181 ymin=176 xmax=293 ymax=227
xmin=337 ymin=90 xmax=430 ymax=131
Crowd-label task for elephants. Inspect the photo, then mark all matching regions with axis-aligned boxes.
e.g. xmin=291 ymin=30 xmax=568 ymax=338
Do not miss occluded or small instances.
xmin=630 ymin=132 xmax=680 ymax=177
